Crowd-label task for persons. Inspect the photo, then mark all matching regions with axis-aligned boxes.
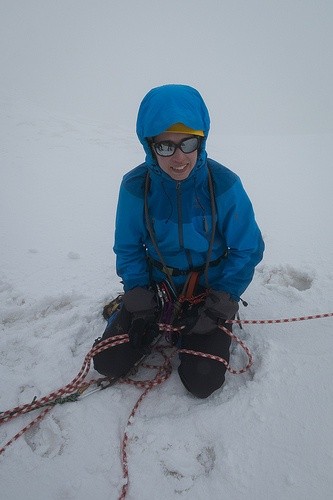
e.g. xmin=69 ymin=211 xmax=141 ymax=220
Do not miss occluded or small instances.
xmin=94 ymin=84 xmax=264 ymax=399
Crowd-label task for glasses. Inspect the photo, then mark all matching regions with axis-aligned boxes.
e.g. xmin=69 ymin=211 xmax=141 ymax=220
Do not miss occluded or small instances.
xmin=151 ymin=136 xmax=201 ymax=157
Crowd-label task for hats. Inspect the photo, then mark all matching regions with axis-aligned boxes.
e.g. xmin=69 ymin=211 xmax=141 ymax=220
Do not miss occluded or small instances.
xmin=164 ymin=124 xmax=205 ymax=137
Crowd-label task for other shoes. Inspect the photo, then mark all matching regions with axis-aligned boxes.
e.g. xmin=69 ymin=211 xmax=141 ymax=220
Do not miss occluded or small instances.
xmin=104 ymin=294 xmax=122 ymax=321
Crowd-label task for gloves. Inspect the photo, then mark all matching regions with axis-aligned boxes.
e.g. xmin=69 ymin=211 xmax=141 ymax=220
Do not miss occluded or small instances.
xmin=178 ymin=288 xmax=239 ymax=344
xmin=122 ymin=285 xmax=160 ymax=356
